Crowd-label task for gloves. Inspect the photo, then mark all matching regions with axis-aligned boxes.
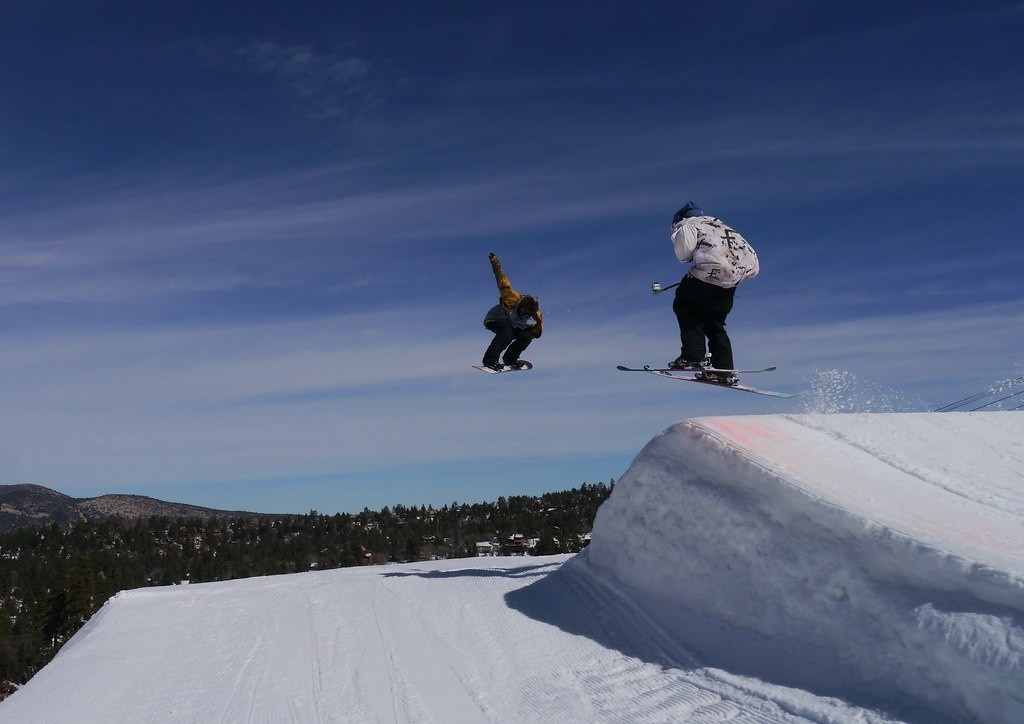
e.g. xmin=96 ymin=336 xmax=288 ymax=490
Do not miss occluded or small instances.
xmin=488 ymin=252 xmax=494 ymax=259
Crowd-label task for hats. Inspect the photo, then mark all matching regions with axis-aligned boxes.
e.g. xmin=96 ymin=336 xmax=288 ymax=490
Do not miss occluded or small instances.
xmin=520 ymin=295 xmax=539 ymax=314
xmin=673 ymin=199 xmax=702 ymax=222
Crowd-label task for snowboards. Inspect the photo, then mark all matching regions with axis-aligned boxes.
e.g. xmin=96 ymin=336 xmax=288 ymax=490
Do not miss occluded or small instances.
xmin=472 ymin=358 xmax=532 ymax=375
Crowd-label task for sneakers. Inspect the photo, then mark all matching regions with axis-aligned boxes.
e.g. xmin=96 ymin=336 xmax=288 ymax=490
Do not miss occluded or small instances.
xmin=668 ymin=351 xmax=711 ymax=371
xmin=504 ymin=358 xmax=521 ymax=370
xmin=482 ymin=356 xmax=503 ymax=371
xmin=694 ymin=368 xmax=740 ymax=387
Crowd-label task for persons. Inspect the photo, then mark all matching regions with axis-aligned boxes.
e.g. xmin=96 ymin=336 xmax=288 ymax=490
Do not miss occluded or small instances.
xmin=483 ymin=253 xmax=542 ymax=372
xmin=667 ymin=202 xmax=760 ymax=384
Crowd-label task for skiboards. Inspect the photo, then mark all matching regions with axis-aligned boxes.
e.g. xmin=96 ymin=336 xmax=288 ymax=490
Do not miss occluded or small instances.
xmin=615 ymin=362 xmax=795 ymax=401
